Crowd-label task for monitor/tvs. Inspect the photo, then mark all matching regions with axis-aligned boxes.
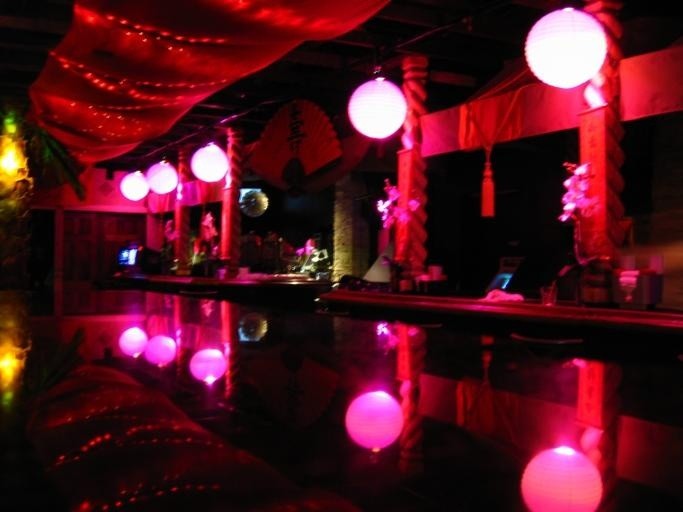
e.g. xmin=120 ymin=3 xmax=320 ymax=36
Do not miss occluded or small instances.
xmin=117 ymin=247 xmax=139 ymax=266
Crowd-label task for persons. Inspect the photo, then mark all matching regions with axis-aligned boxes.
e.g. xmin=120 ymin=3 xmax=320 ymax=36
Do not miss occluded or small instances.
xmin=232 ymin=333 xmax=342 ymax=485
